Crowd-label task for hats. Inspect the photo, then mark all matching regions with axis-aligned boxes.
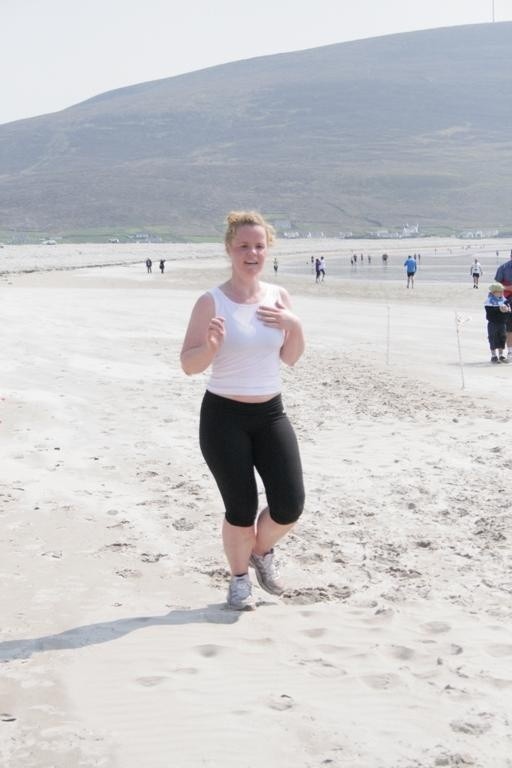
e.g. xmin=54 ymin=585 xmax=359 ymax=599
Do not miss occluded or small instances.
xmin=489 ymin=282 xmax=504 ymax=292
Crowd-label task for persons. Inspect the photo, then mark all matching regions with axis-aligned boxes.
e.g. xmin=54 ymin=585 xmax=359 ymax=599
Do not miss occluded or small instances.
xmin=471 ymin=259 xmax=482 ymax=289
xmin=382 ymin=253 xmax=389 ymax=264
xmin=414 ymin=253 xmax=416 ymax=260
xmin=273 ymin=256 xmax=279 ymax=271
xmin=315 ymin=259 xmax=321 ymax=282
xmin=146 ymin=257 xmax=153 ymax=273
xmin=311 ymin=255 xmax=314 ymax=263
xmin=350 ymin=250 xmax=371 ymax=265
xmin=159 ymin=258 xmax=167 ymax=273
xmin=485 ymin=283 xmax=510 ymax=362
xmin=319 ymin=256 xmax=327 ymax=280
xmin=494 ymin=249 xmax=511 ymax=361
xmin=179 ymin=209 xmax=312 ymax=610
xmin=404 ymin=256 xmax=417 ymax=289
xmin=417 ymin=252 xmax=421 ymax=261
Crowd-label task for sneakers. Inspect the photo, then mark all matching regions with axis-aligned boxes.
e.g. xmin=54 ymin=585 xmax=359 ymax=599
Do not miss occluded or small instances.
xmin=491 ymin=352 xmax=512 ymax=364
xmin=249 ymin=549 xmax=286 ymax=595
xmin=228 ymin=576 xmax=256 ymax=611
